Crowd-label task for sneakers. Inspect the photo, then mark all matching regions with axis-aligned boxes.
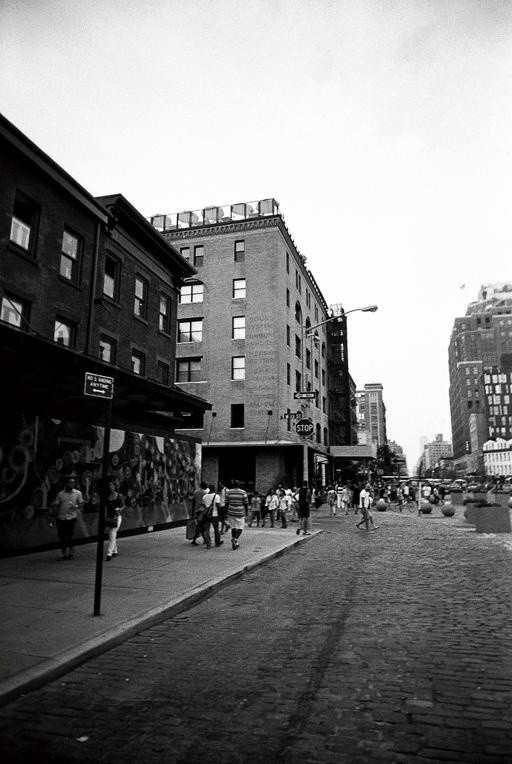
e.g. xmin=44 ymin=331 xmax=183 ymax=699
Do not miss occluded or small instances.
xmin=231 ymin=537 xmax=239 ymax=549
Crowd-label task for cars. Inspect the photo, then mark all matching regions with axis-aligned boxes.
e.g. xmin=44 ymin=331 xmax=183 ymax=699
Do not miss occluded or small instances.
xmin=379 ymin=475 xmax=511 ymax=493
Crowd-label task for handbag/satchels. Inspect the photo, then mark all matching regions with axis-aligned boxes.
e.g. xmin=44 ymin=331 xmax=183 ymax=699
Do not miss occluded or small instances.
xmin=186 ymin=517 xmax=199 ymax=540
xmin=202 ymin=493 xmax=216 ymax=520
xmin=104 ymin=516 xmax=117 ymax=527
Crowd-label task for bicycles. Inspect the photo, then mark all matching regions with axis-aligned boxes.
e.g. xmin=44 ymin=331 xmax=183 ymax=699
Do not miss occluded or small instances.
xmin=388 ymin=492 xmax=416 ymax=514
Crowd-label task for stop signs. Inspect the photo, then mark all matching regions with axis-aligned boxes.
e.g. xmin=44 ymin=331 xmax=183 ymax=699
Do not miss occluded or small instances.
xmin=294 ymin=418 xmax=316 ymax=438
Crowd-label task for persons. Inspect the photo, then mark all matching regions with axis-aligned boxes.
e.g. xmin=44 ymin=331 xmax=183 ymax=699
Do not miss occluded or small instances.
xmin=49 ymin=476 xmax=85 ymax=560
xmin=190 ymin=476 xmax=445 ymax=550
xmin=104 ymin=481 xmax=126 ymax=562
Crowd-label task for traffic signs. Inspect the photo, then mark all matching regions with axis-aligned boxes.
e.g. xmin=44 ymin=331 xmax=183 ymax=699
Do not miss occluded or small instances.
xmin=293 ymin=391 xmax=316 ymax=399
xmin=83 ymin=371 xmax=113 ymax=400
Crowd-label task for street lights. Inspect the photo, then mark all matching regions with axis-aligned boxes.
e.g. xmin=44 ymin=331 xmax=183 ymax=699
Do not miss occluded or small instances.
xmin=296 ymin=303 xmax=378 ymax=533
